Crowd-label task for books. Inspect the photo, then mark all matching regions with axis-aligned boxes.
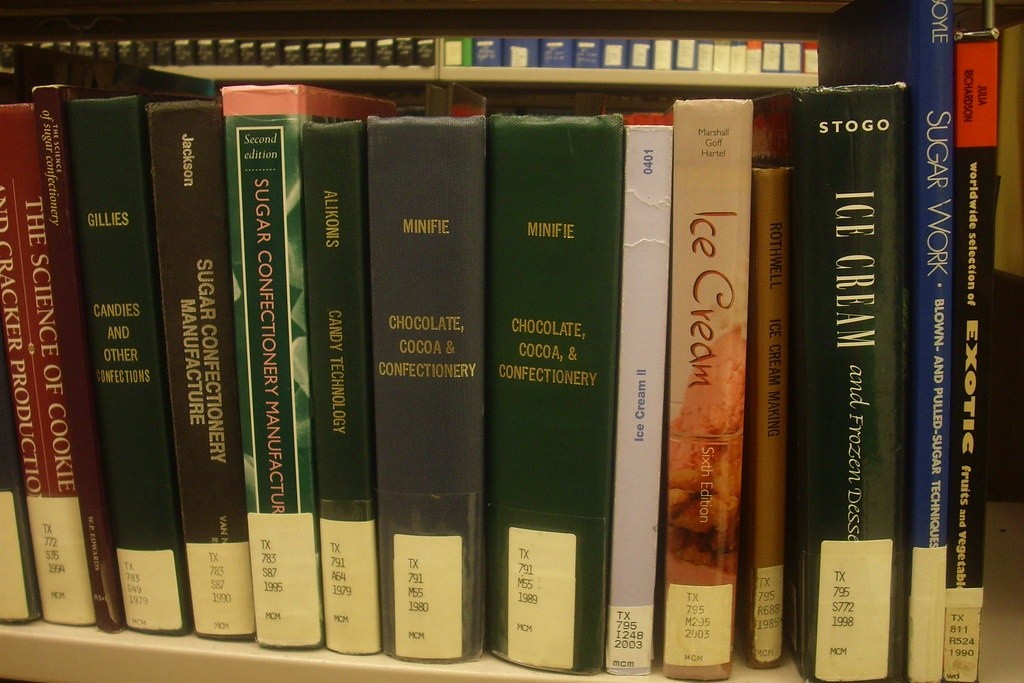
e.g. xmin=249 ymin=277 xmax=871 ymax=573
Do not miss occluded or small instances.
xmin=0 ymin=2 xmax=1007 ymax=680
